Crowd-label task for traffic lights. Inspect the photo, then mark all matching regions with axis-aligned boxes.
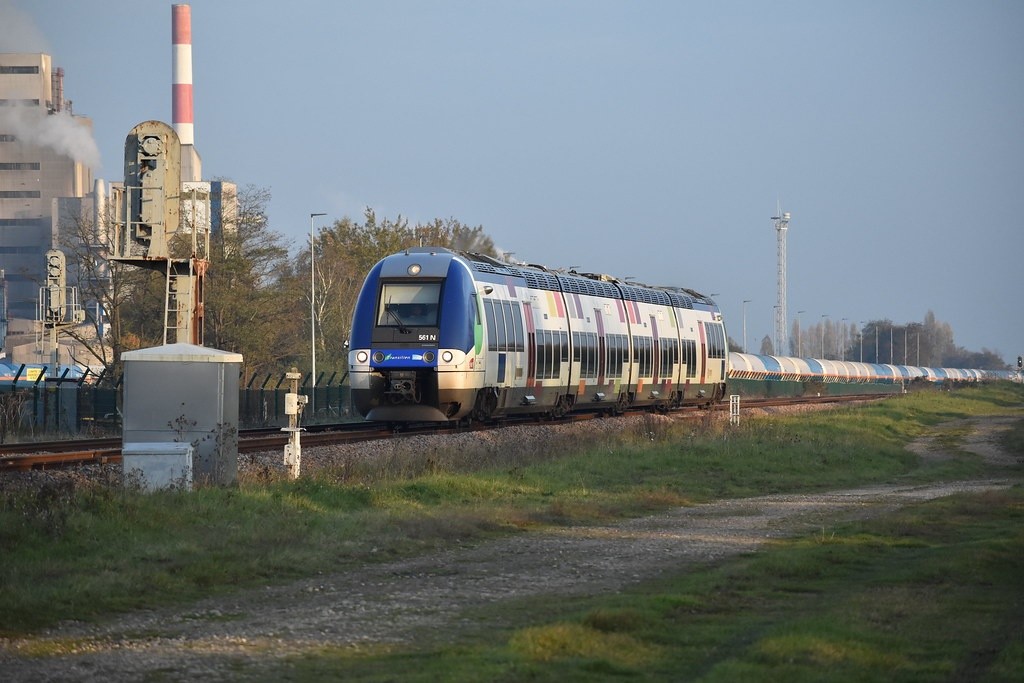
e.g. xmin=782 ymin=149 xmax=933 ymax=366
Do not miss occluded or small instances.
xmin=1018 ymin=356 xmax=1022 ymax=367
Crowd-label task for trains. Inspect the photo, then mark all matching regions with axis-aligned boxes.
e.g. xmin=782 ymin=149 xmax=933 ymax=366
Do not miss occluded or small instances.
xmin=338 ymin=237 xmax=730 ymax=432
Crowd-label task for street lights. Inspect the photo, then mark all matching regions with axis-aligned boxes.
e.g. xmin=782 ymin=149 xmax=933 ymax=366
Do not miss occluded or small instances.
xmin=309 ymin=213 xmax=329 ymax=412
xmin=742 ymin=300 xmax=753 ymax=352
xmin=842 ymin=318 xmax=847 ymax=361
xmin=875 ymin=325 xmax=880 ymax=364
xmin=821 ymin=314 xmax=830 ymax=358
xmin=798 ymin=310 xmax=806 ymax=357
xmin=860 ymin=320 xmax=866 ymax=360
xmin=772 ymin=305 xmax=781 ymax=356
